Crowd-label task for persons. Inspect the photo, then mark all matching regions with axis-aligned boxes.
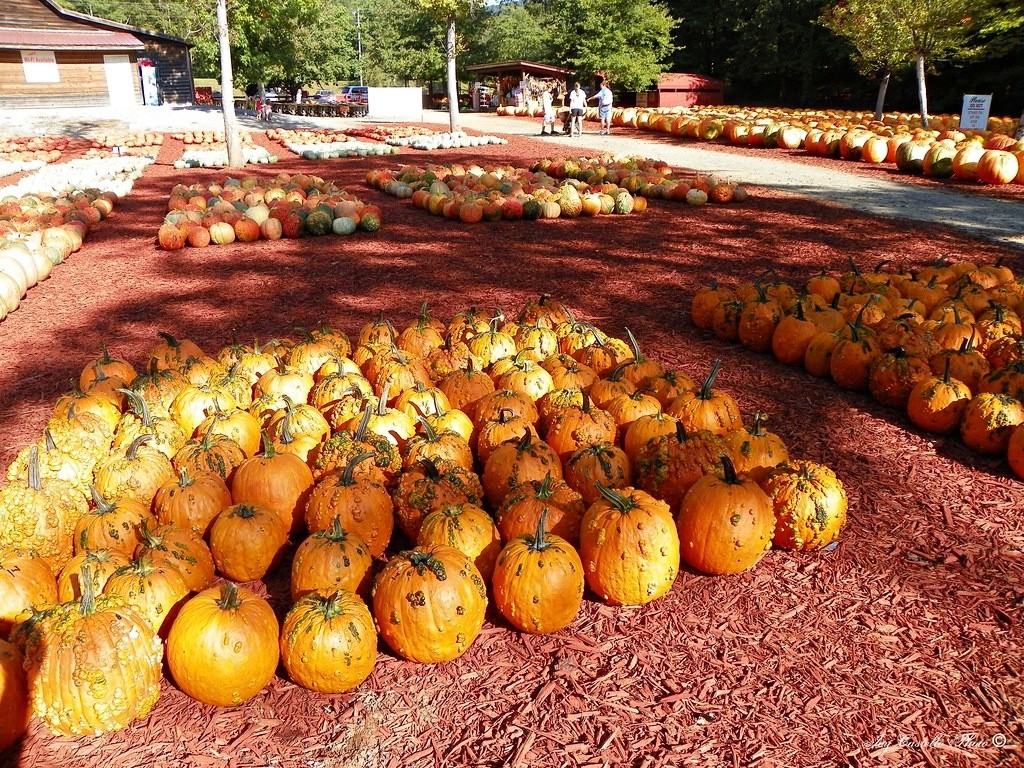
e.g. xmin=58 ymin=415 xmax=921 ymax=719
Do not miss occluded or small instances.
xmin=569 ymin=82 xmax=587 ymax=138
xmin=296 ymin=89 xmax=302 ymax=104
xmin=587 ymin=82 xmax=613 ymax=135
xmin=256 ymin=98 xmax=272 ymax=122
xmin=541 ymin=87 xmax=559 ymax=135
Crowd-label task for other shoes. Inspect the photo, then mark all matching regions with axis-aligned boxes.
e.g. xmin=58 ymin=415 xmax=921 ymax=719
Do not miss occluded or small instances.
xmin=600 ymin=131 xmax=604 ymax=135
xmin=542 ymin=131 xmax=549 ymax=135
xmin=551 ymin=131 xmax=559 ymax=136
xmin=607 ymin=130 xmax=610 ymax=135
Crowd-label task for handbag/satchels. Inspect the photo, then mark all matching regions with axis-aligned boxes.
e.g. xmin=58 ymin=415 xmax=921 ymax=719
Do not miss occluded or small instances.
xmin=602 ymin=96 xmax=611 ymax=106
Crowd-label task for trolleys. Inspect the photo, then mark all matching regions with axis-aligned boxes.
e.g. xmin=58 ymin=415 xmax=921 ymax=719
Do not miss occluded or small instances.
xmin=558 ymin=111 xmax=587 ymax=135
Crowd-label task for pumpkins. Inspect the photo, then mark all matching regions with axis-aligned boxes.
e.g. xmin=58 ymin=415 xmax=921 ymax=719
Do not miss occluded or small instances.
xmin=0 ymin=292 xmax=848 ymax=754
xmin=170 ymin=126 xmax=508 ymax=169
xmin=692 ymin=253 xmax=1024 ymax=483
xmin=0 ymin=133 xmax=166 ymax=320
xmin=497 ymin=103 xmax=1024 ymax=184
xmin=158 ymin=153 xmax=749 ymax=249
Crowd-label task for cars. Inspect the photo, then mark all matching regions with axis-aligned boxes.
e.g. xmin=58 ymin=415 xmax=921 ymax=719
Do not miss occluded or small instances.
xmin=264 ymin=87 xmax=293 ymax=105
xmin=316 ymin=90 xmax=338 ymax=103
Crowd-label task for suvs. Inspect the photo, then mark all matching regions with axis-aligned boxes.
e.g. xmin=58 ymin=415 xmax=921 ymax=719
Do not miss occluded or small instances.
xmin=342 ymin=86 xmax=368 ymax=103
